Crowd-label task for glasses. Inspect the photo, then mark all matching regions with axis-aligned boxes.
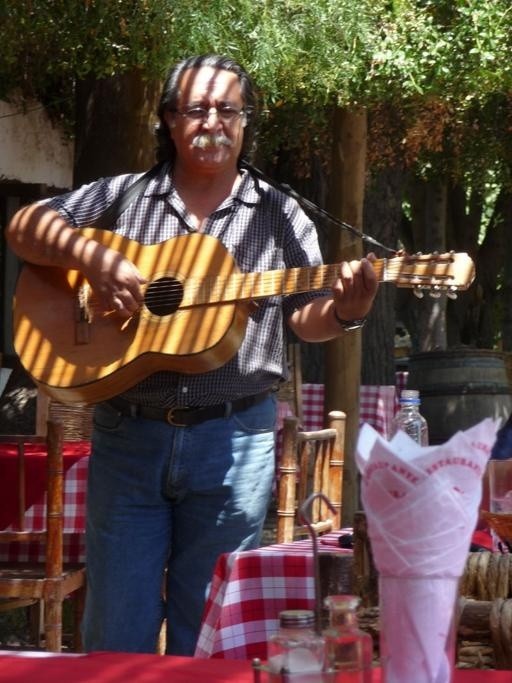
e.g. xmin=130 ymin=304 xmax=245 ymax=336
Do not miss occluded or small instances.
xmin=173 ymin=105 xmax=248 ymax=123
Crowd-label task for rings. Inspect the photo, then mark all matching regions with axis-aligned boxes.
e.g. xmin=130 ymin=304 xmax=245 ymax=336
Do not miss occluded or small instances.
xmin=111 ymin=297 xmax=122 ymax=311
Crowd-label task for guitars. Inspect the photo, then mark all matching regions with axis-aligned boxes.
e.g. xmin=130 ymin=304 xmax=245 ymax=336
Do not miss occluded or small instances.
xmin=11 ymin=227 xmax=477 ymax=409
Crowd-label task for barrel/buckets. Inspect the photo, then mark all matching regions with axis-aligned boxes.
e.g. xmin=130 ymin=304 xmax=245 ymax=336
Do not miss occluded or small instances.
xmin=407 ymin=350 xmax=511 ymax=449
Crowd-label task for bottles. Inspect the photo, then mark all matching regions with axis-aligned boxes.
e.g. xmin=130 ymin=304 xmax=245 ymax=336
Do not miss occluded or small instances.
xmin=388 ymin=388 xmax=428 ymax=447
xmin=268 ymin=594 xmax=373 ymax=683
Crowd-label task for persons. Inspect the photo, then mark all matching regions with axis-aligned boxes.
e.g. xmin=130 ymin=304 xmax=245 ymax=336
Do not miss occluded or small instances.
xmin=6 ymin=54 xmax=378 ymax=657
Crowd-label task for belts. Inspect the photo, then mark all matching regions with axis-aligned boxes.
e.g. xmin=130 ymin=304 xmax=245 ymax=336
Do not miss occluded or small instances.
xmin=110 ymin=390 xmax=273 ymax=428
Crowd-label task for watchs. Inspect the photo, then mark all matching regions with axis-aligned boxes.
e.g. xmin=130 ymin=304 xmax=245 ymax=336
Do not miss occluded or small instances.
xmin=333 ymin=311 xmax=369 ymax=333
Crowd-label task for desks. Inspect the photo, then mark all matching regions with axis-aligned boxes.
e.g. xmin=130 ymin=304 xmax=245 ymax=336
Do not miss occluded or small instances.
xmin=194 ymin=526 xmax=354 ymax=661
xmin=274 ymin=384 xmax=401 ymax=497
xmin=0 ymin=441 xmax=92 ymax=562
xmin=0 ymin=651 xmax=512 ymax=681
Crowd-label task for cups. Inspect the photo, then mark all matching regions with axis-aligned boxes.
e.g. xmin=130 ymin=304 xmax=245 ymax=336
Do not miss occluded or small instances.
xmin=379 ymin=575 xmax=461 ymax=682
